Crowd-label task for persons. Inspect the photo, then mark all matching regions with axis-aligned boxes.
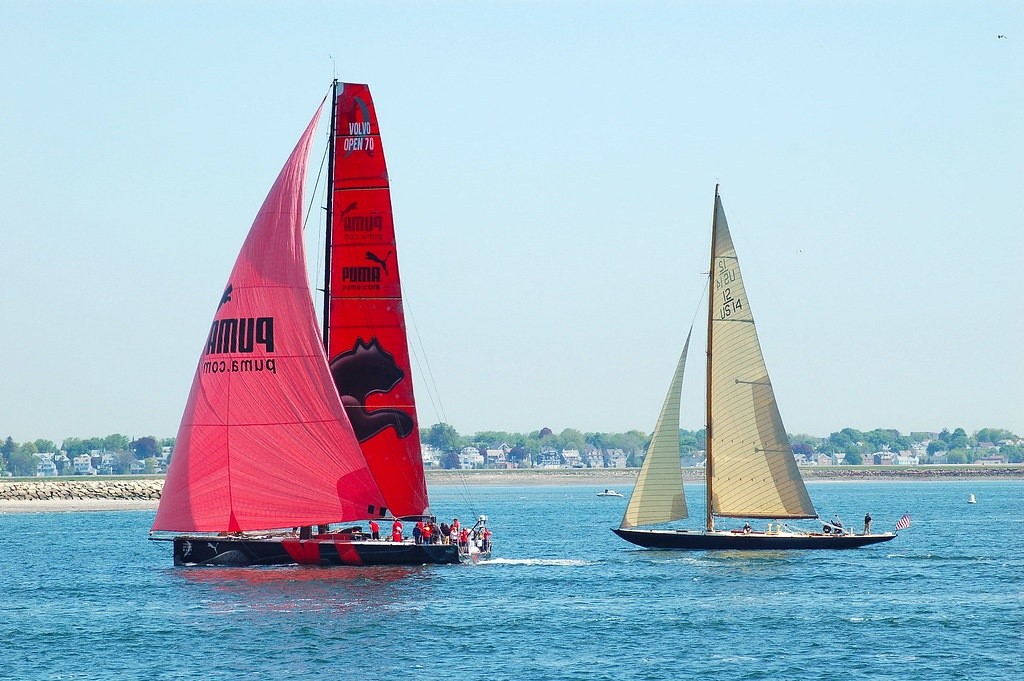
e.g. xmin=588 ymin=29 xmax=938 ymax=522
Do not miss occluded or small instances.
xmin=292 ymin=527 xmax=298 ymax=538
xmin=369 ymin=520 xmax=380 ymax=540
xmin=743 ymin=523 xmax=751 ymax=535
xmin=830 ymin=518 xmax=843 ymax=535
xmin=863 ymin=513 xmax=871 ymax=535
xmin=392 ymin=518 xmax=492 ymax=553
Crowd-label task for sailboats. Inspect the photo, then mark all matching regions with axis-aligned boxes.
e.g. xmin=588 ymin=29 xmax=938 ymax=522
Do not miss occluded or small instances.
xmin=147 ymin=52 xmax=494 ymax=569
xmin=607 ymin=177 xmax=912 ymax=554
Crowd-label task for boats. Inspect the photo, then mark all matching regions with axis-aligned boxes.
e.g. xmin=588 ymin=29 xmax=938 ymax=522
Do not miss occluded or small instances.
xmin=597 ymin=488 xmax=624 ymax=498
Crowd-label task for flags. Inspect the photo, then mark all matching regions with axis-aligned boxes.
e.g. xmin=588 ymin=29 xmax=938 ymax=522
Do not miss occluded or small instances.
xmin=895 ymin=514 xmax=911 ymax=531
